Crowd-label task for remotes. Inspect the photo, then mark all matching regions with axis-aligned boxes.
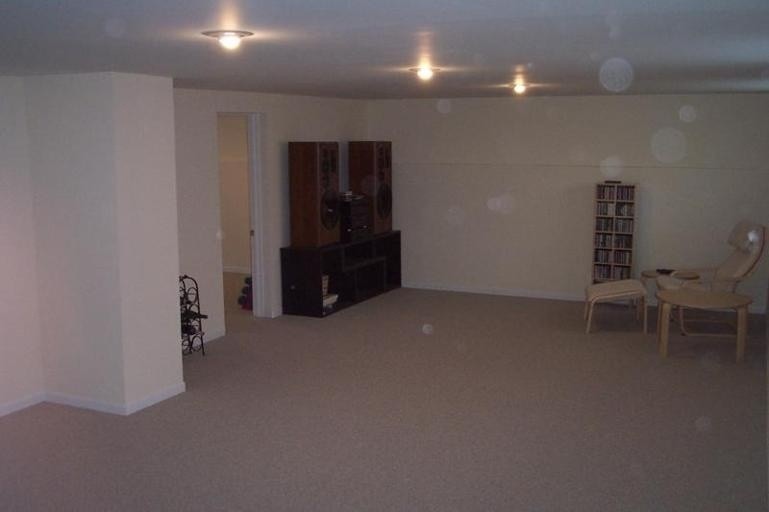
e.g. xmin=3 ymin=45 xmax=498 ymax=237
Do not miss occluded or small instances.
xmin=604 ymin=180 xmax=621 ymax=184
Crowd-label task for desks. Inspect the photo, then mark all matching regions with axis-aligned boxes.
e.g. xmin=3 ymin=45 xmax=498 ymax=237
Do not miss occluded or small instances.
xmin=651 ymin=287 xmax=755 ymax=365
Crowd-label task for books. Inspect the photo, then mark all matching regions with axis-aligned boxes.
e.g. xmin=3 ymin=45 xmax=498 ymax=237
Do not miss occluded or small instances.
xmin=593 ymin=180 xmax=634 ymax=280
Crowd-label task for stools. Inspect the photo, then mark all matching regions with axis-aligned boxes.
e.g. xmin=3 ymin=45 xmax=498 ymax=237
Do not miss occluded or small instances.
xmin=642 ymin=269 xmax=701 ymax=330
xmin=583 ymin=278 xmax=648 ymax=336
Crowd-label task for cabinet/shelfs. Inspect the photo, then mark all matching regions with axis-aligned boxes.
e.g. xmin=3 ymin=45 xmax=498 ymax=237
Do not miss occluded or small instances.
xmin=279 ymin=232 xmax=404 ymax=320
xmin=591 ymin=179 xmax=636 ymax=292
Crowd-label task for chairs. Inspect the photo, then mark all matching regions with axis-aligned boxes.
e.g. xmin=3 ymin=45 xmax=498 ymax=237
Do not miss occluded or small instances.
xmin=655 ymin=220 xmax=768 ymax=337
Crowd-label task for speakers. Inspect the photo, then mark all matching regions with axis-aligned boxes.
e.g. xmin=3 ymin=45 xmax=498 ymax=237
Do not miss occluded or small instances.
xmin=340 ymin=193 xmax=370 ymax=243
xmin=349 ymin=140 xmax=393 ymax=233
xmin=287 ymin=141 xmax=341 ymax=250
